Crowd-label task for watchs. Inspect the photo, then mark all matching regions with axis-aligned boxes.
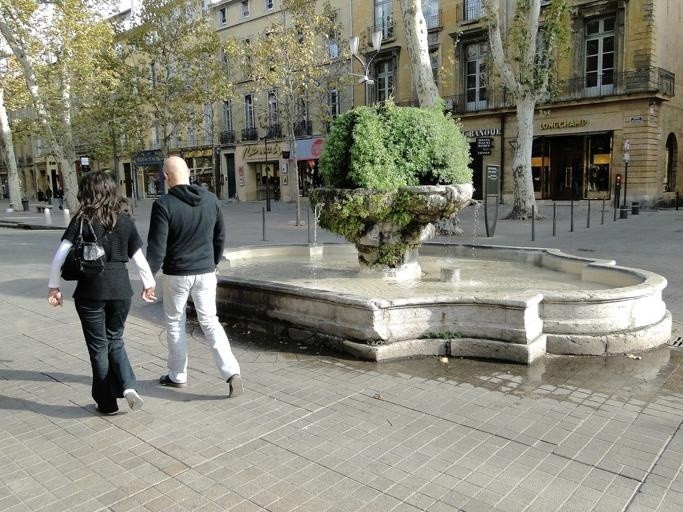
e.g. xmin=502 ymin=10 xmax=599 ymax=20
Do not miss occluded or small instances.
xmin=48 ymin=288 xmax=60 ymax=292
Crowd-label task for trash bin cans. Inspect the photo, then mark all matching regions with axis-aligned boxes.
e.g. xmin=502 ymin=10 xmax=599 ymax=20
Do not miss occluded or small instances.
xmin=22 ymin=200 xmax=28 ymax=210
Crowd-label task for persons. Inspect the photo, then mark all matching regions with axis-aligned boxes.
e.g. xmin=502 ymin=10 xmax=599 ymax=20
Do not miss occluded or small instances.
xmin=262 ymin=167 xmax=274 ymax=185
xmin=48 ymin=171 xmax=157 ymax=415
xmin=37 ymin=184 xmax=64 ymax=210
xmin=142 ymin=156 xmax=243 ymax=398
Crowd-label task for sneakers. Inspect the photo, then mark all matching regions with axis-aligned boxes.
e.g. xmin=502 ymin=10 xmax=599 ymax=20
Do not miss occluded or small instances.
xmin=95 ymin=388 xmax=144 ymax=415
xmin=160 ymin=374 xmax=187 ymax=388
xmin=227 ymin=373 xmax=244 ymax=398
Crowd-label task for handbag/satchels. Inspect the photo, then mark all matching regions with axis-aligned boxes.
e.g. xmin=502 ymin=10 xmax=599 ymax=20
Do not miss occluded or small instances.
xmin=61 ymin=215 xmax=106 ymax=281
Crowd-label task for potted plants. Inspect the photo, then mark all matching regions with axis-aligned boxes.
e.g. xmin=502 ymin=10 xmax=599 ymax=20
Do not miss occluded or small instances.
xmin=307 ymin=98 xmax=475 ymax=265
